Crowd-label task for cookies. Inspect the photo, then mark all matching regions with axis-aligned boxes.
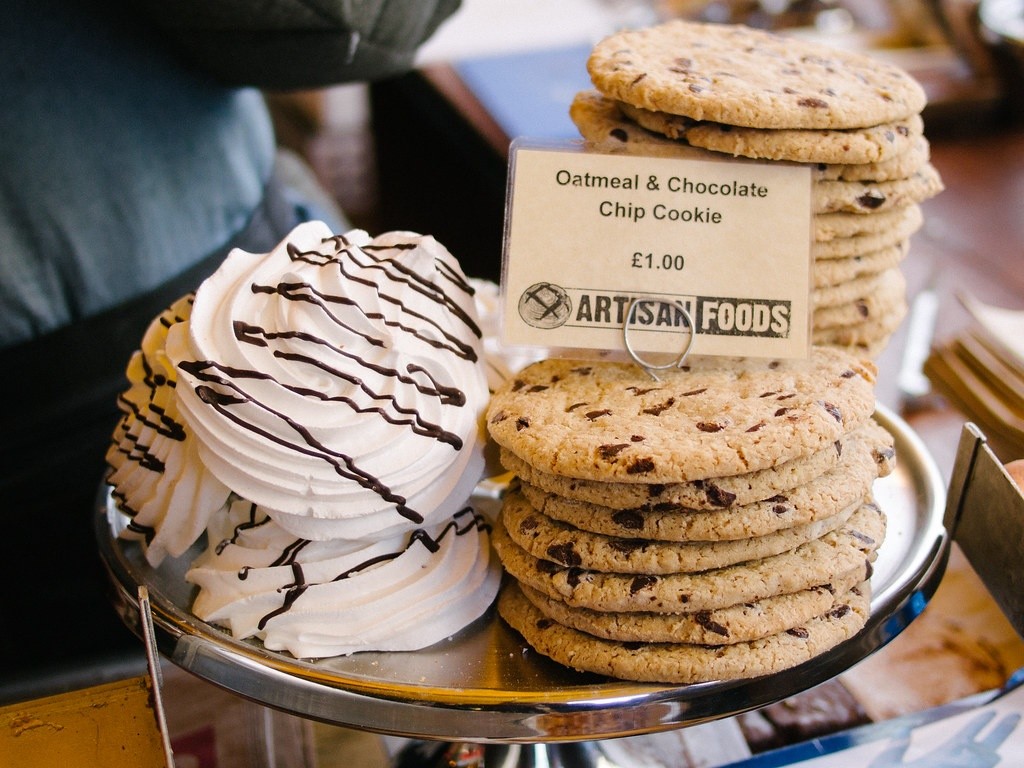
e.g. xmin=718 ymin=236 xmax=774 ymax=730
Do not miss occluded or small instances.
xmin=569 ymin=20 xmax=942 ymax=355
xmin=486 ymin=355 xmax=897 ymax=679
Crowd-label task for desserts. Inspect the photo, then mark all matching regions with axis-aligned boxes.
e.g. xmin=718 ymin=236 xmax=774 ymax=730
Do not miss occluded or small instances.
xmin=105 ymin=219 xmax=543 ymax=660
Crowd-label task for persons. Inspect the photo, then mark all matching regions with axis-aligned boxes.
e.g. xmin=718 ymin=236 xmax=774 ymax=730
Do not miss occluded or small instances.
xmin=0 ymin=0 xmax=464 ymax=706
